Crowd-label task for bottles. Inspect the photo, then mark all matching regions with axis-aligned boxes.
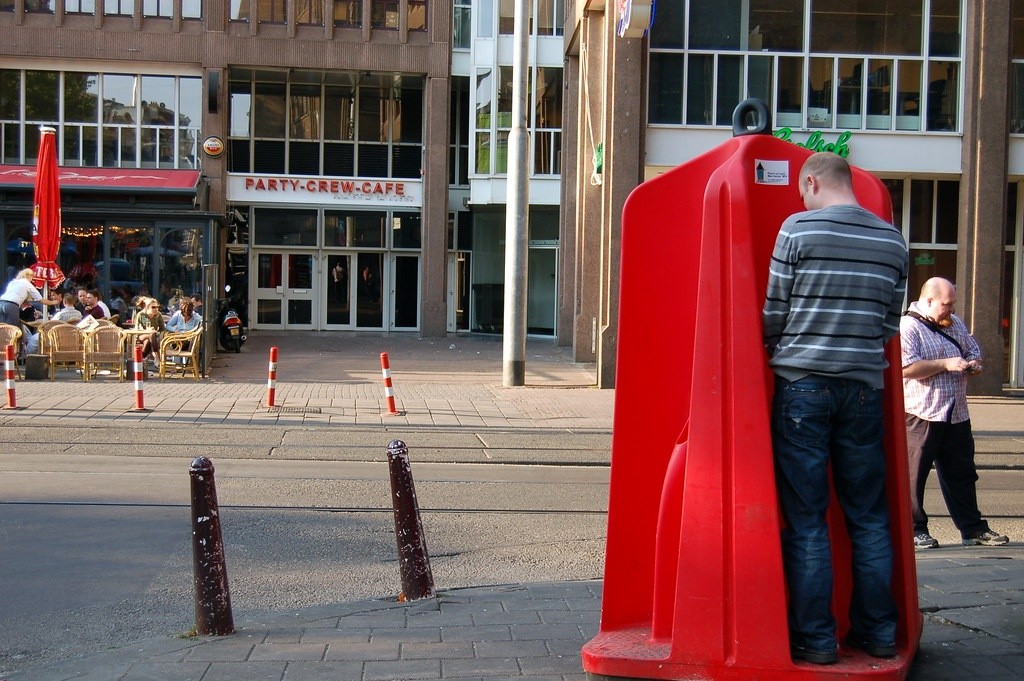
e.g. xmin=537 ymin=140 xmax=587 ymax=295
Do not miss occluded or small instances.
xmin=132 ymin=309 xmax=137 ymax=324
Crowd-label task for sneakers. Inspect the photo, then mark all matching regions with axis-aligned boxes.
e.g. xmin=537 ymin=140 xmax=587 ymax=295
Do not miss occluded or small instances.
xmin=962 ymin=527 xmax=1009 ymax=545
xmin=913 ymin=533 xmax=938 ymax=549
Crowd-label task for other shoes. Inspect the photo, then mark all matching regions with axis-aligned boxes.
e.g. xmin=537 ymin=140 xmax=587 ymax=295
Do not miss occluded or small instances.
xmin=154 ymin=361 xmax=160 ymax=369
xmin=791 ymin=642 xmax=838 ymax=663
xmin=842 ymin=628 xmax=896 ymax=657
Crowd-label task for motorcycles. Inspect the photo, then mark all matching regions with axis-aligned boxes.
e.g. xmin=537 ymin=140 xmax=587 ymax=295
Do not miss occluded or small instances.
xmin=212 ymin=284 xmax=247 ymax=353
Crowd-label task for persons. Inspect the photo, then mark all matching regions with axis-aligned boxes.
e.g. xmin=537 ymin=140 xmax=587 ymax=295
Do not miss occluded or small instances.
xmin=167 ymin=289 xmax=184 ymax=318
xmin=900 ymin=277 xmax=1009 ymax=549
xmin=166 ymin=297 xmax=202 ymax=373
xmin=74 ymin=288 xmax=88 ymax=319
xmin=191 ymin=294 xmax=202 ymax=316
xmin=135 ymin=298 xmax=165 ymax=368
xmin=25 ymin=293 xmax=82 ymax=356
xmin=763 ymin=151 xmax=909 ymax=663
xmin=77 ymin=290 xmax=111 ymax=334
xmin=18 ymin=296 xmax=35 ymax=359
xmin=49 ymin=289 xmax=65 ymax=316
xmin=109 ymin=289 xmax=127 ymax=325
xmin=135 ymin=296 xmax=148 ymax=323
xmin=0 ymin=268 xmax=59 ymax=326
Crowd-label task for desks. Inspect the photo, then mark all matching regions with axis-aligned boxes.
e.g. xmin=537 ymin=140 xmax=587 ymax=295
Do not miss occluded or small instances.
xmin=124 ymin=329 xmax=157 ymax=358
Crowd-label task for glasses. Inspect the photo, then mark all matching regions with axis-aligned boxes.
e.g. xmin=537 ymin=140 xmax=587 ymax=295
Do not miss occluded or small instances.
xmin=150 ymin=305 xmax=160 ymax=309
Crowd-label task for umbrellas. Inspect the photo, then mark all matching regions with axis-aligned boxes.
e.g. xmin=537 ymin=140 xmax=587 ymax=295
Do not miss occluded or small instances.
xmin=30 ymin=126 xmax=66 ymax=323
xmin=68 ymin=236 xmax=97 ymax=292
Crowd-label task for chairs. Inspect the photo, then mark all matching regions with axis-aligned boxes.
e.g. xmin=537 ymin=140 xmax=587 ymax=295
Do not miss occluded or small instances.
xmin=0 ymin=317 xmax=205 ymax=382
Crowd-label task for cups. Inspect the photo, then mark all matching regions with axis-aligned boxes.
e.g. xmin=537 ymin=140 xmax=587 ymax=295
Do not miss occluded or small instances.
xmin=146 ymin=322 xmax=151 ymax=332
